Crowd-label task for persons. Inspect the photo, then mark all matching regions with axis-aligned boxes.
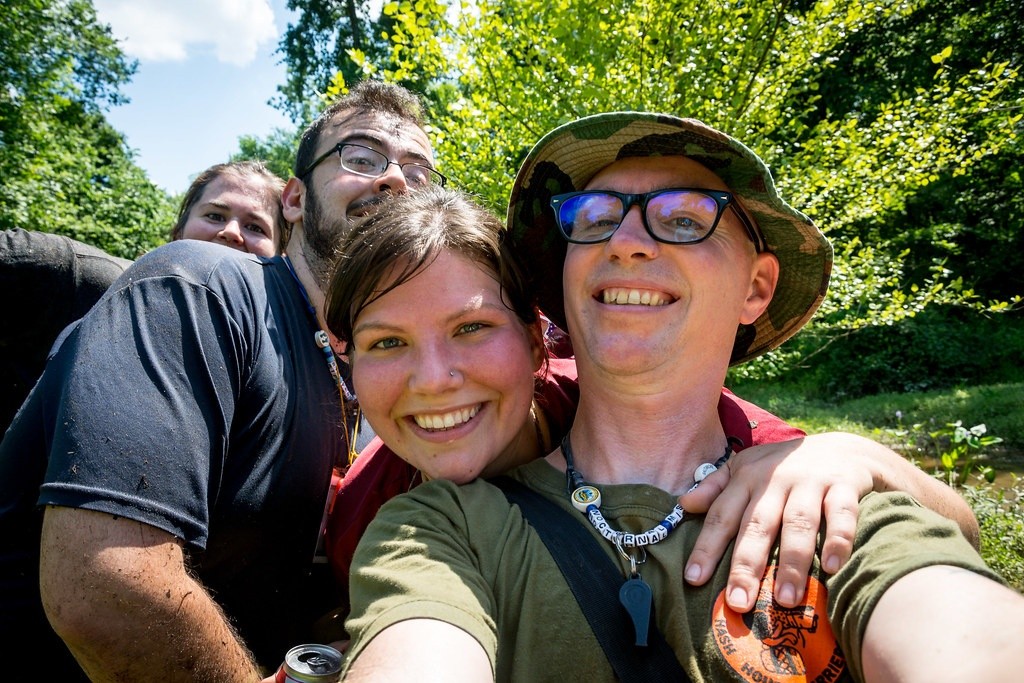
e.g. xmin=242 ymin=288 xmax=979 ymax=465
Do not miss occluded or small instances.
xmin=0 ymin=82 xmax=447 ymax=683
xmin=339 ymin=112 xmax=1024 ymax=683
xmin=324 ymin=187 xmax=987 ymax=648
xmin=173 ymin=161 xmax=289 ymax=262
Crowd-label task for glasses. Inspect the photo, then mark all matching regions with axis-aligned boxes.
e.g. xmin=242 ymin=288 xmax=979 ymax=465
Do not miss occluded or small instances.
xmin=305 ymin=142 xmax=446 ymax=195
xmin=550 ymin=188 xmax=762 ymax=256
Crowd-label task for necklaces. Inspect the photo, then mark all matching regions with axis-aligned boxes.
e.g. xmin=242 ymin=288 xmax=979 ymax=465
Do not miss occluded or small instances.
xmin=278 ymin=253 xmax=362 ymax=509
xmin=557 ymin=428 xmax=735 ymax=646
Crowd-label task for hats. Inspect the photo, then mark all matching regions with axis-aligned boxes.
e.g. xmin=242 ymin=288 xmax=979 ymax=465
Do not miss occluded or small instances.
xmin=507 ymin=112 xmax=835 ymax=366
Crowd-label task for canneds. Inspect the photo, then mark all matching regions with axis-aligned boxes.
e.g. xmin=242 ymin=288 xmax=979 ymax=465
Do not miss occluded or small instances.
xmin=275 ymin=644 xmax=343 ymax=683
